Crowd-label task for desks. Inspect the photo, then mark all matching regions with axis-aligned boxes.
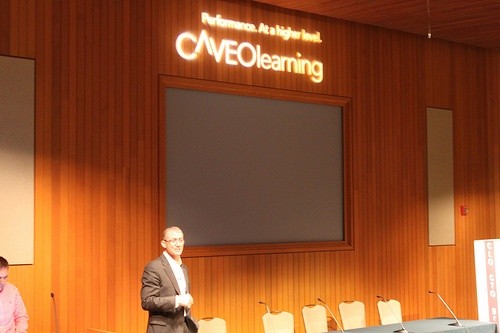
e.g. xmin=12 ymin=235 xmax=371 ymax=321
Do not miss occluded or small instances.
xmin=329 ymin=317 xmax=498 ymax=333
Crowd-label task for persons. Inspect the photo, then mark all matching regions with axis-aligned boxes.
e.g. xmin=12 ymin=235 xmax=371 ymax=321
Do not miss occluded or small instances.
xmin=141 ymin=227 xmax=198 ymax=333
xmin=0 ymin=256 xmax=29 ymax=333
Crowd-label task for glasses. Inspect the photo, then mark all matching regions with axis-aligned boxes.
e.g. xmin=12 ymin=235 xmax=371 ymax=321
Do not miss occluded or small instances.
xmin=162 ymin=239 xmax=184 ymax=244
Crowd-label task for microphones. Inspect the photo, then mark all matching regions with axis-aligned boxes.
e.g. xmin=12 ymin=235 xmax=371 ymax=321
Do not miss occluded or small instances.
xmin=318 ymin=298 xmax=345 ymax=333
xmin=51 ymin=292 xmax=57 ymax=318
xmin=428 ymin=291 xmax=463 ymax=326
xmin=376 ymin=295 xmax=408 ymax=333
xmin=258 ymin=301 xmax=270 ymax=313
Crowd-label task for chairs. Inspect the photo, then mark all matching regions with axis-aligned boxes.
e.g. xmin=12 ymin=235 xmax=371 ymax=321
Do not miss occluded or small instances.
xmin=263 ymin=312 xmax=295 ymax=333
xmin=301 ymin=304 xmax=329 ymax=333
xmin=339 ymin=301 xmax=365 ymax=330
xmin=377 ymin=299 xmax=403 ymax=325
xmin=198 ymin=317 xmax=227 ymax=333
